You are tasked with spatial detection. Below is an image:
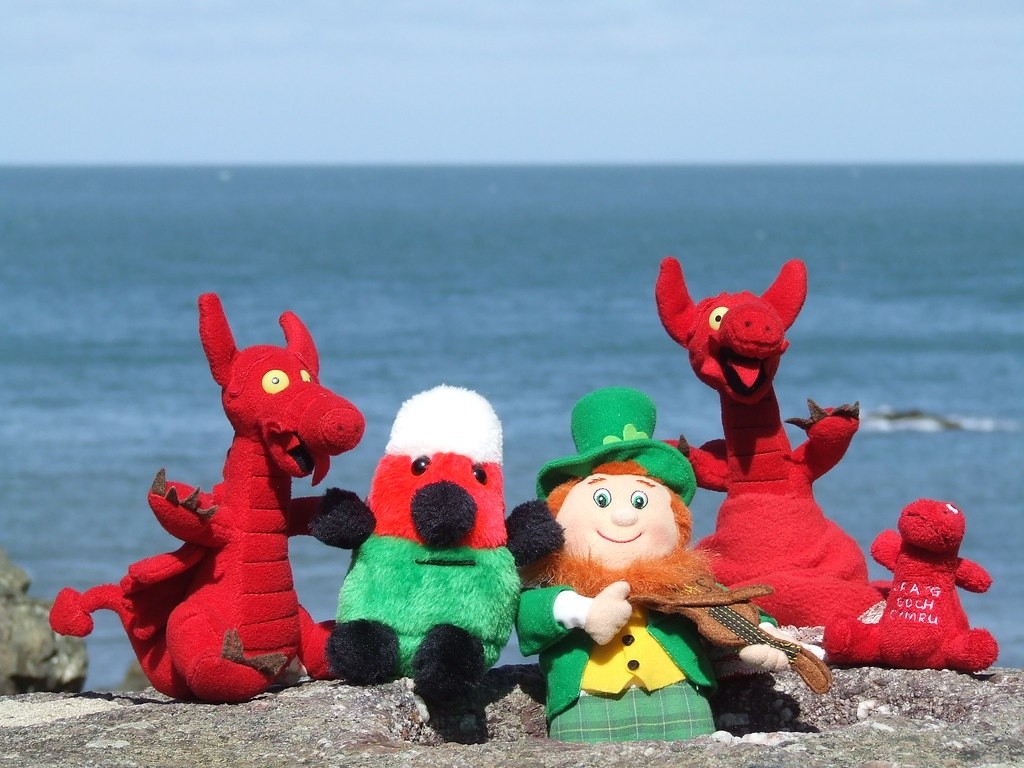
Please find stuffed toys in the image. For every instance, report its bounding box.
[50,293,365,701]
[513,387,831,741]
[655,257,998,672]
[308,383,564,746]
